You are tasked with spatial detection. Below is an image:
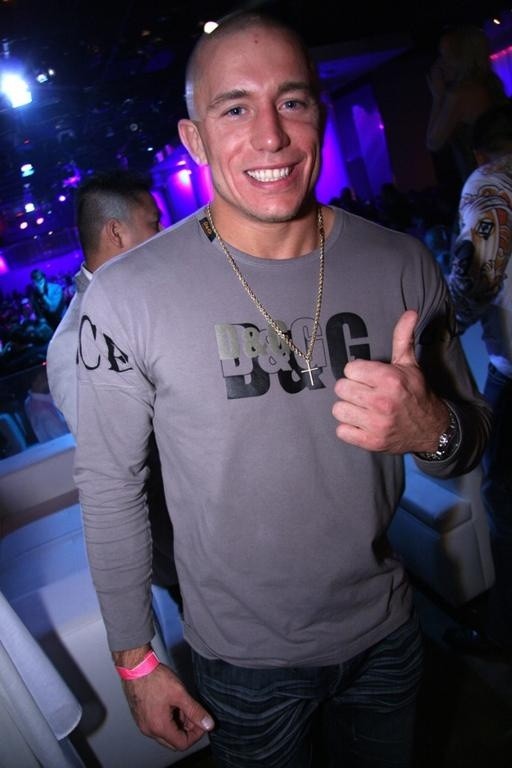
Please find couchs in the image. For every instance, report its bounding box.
[1,429,496,768]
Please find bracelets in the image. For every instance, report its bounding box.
[114,651,161,681]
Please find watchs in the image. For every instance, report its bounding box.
[417,402,460,462]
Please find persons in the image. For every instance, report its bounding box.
[423,21,507,225]
[0,267,78,461]
[444,102,512,662]
[45,169,184,619]
[330,181,453,274]
[71,7,494,767]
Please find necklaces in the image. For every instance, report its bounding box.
[206,202,326,387]
